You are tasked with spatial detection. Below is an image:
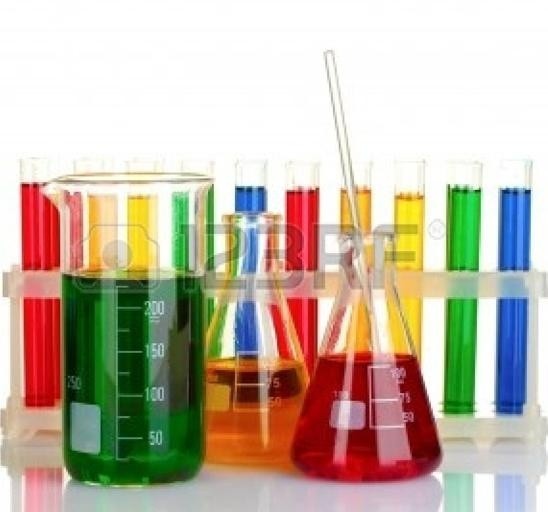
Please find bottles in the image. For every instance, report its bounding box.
[21,157,532,486]
